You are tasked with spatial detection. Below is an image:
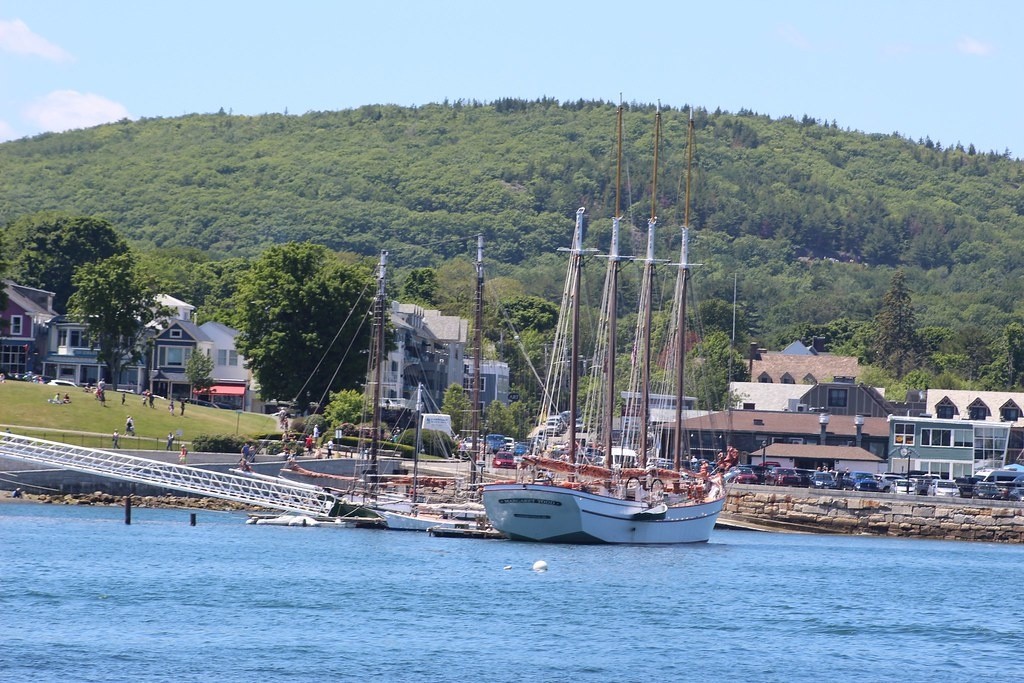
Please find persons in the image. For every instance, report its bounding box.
[142,389,155,409]
[112,429,121,449]
[0,373,5,381]
[392,432,398,442]
[279,406,288,430]
[168,399,186,416]
[94,378,106,406]
[241,443,255,463]
[14,371,33,382]
[814,466,850,479]
[281,424,321,461]
[179,445,188,464]
[121,391,126,405]
[166,431,174,451]
[54,392,70,404]
[123,415,136,437]
[327,438,333,459]
[691,455,697,462]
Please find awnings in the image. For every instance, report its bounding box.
[193,386,246,397]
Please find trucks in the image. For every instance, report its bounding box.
[973,468,1024,482]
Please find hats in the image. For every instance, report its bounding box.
[114,428,118,431]
[181,445,185,447]
[127,415,130,417]
[315,425,319,427]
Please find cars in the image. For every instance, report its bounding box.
[973,482,1024,501]
[844,471,877,491]
[486,411,601,468]
[727,465,846,490]
[48,380,78,387]
[955,477,977,498]
[647,457,716,473]
[907,474,941,494]
[873,474,905,493]
[890,479,917,495]
[927,479,961,497]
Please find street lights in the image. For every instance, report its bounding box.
[906,450,912,495]
[762,440,766,484]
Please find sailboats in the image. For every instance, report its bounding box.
[338,381,494,533]
[227,233,486,519]
[482,91,725,546]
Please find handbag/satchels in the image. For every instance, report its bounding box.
[127,423,131,427]
